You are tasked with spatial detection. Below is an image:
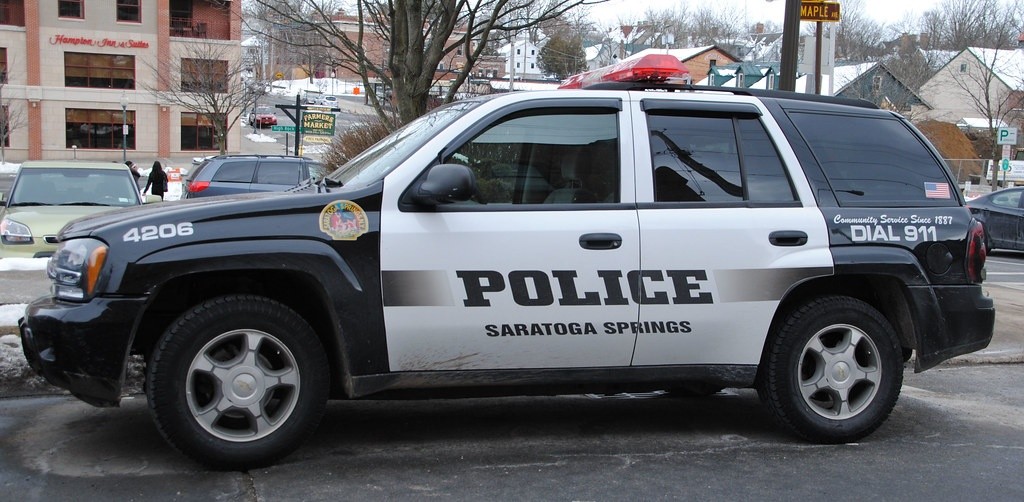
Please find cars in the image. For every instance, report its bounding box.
[320,96,339,112]
[249,106,277,128]
[0,159,162,255]
[964,187,1024,254]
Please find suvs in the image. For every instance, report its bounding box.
[10,49,996,445]
[179,155,334,199]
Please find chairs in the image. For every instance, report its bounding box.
[91,183,112,198]
[65,188,85,197]
[39,180,56,196]
[543,147,596,204]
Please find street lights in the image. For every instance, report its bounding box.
[121,90,129,162]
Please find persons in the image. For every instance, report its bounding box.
[141,160,168,201]
[125,160,140,195]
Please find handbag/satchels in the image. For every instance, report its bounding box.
[163,171,167,192]
